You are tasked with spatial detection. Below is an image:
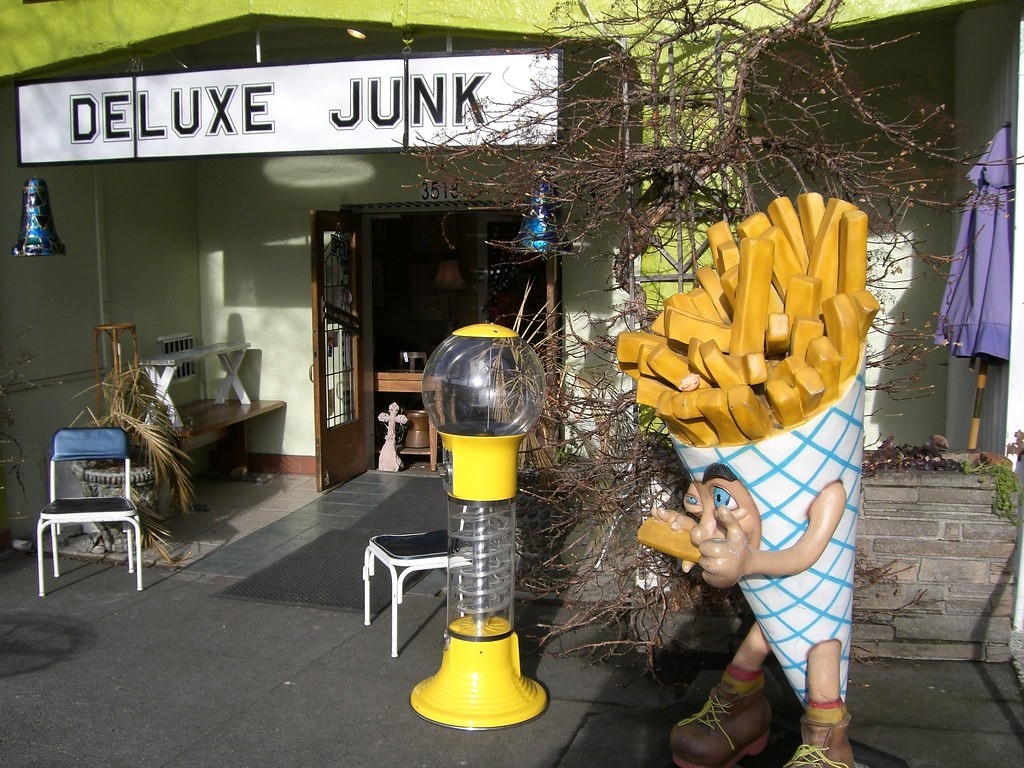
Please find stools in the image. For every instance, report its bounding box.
[400,351,428,372]
[94,322,137,417]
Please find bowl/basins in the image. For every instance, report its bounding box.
[405,409,429,431]
[404,431,432,448]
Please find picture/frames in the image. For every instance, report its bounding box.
[409,258,452,322]
[409,214,462,256]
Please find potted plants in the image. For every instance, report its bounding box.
[61,362,198,567]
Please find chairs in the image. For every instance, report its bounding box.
[361,506,467,658]
[36,427,144,597]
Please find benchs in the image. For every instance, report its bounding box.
[137,342,251,427]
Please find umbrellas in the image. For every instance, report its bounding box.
[932,122,1012,455]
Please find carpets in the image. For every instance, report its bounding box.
[208,478,446,615]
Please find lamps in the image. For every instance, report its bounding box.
[514,159,576,256]
[430,259,468,335]
[11,168,68,258]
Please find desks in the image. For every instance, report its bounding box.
[375,369,441,471]
[171,399,285,513]
